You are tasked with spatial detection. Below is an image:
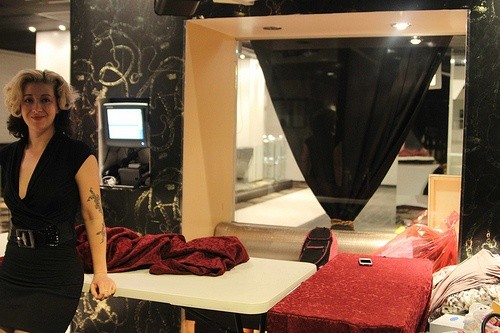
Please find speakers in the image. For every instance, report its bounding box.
[153,0,202,17]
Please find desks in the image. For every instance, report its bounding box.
[268,252,434,332]
[83,255,317,333]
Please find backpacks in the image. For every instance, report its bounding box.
[300,227,339,269]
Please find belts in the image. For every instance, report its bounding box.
[6,225,75,249]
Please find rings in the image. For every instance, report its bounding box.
[104,290,108,292]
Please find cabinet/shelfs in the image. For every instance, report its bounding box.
[395,156,440,209]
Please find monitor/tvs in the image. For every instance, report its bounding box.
[103,102,148,148]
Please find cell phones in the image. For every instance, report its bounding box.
[359,258,372,265]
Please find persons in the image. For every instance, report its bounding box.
[0,67,117,333]
